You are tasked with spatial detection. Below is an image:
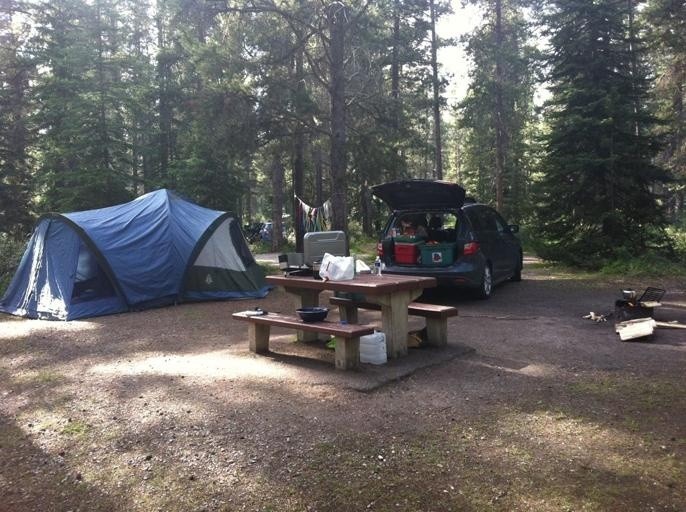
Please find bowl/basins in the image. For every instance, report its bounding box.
[296,307,329,322]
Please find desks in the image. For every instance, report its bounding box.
[266,272,437,358]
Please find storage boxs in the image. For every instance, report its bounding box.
[394,240,426,263]
[418,242,456,266]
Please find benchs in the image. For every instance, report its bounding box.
[329,296,458,348]
[232,311,374,371]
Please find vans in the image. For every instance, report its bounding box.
[370,180,524,299]
[249,222,287,245]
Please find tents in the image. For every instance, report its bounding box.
[0,187,272,320]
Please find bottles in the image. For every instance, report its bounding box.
[374,255,382,278]
